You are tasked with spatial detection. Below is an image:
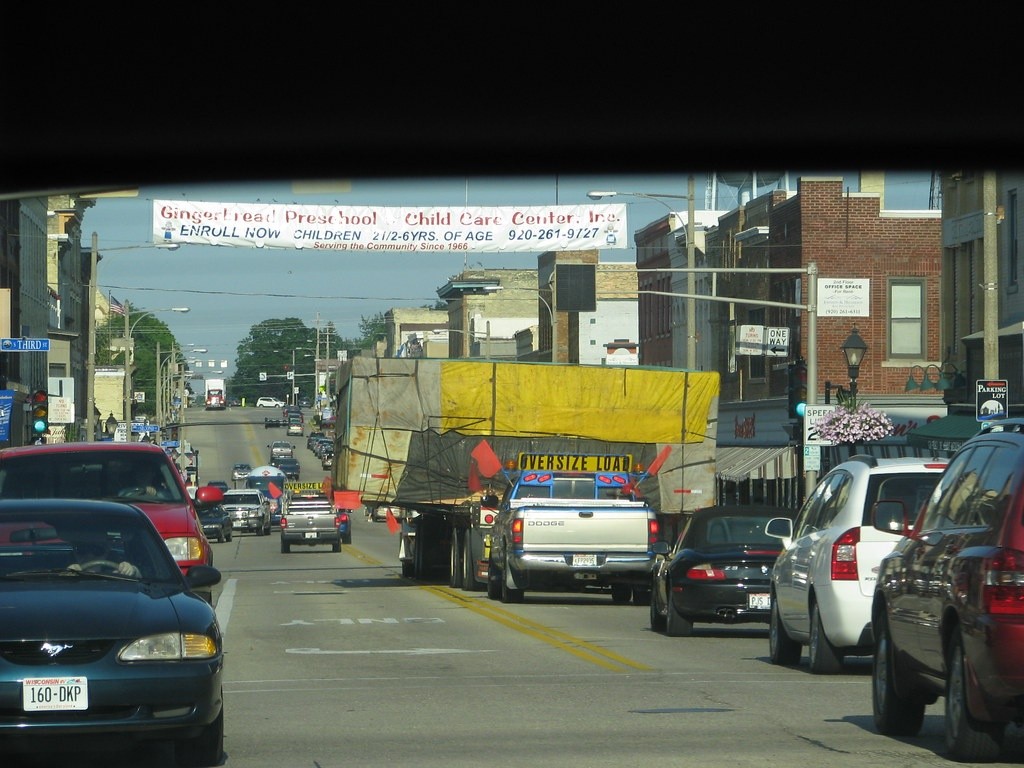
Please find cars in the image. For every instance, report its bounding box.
[283,405,304,436]
[298,396,312,409]
[231,463,252,481]
[0,441,224,768]
[280,490,354,554]
[306,432,336,470]
[650,505,799,637]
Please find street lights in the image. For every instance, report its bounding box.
[482,264,556,361]
[433,319,491,360]
[274,322,329,408]
[86,230,180,442]
[155,341,208,425]
[586,177,696,371]
[124,300,190,443]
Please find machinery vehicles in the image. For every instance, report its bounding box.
[244,466,289,522]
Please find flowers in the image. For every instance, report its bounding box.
[814,390,895,446]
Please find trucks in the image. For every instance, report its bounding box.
[398,503,501,597]
[204,378,227,410]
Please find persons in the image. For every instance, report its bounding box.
[118,457,156,497]
[140,432,158,445]
[64,529,142,580]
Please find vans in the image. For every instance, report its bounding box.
[267,440,301,480]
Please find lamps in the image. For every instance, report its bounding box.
[920,364,954,391]
[937,363,961,390]
[904,365,940,392]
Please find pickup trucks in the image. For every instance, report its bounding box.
[480,469,660,603]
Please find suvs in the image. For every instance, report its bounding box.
[765,454,949,676]
[193,488,272,543]
[871,417,1024,761]
[256,396,286,408]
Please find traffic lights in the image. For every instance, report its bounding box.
[30,387,49,434]
[170,423,179,441]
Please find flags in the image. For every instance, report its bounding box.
[646,445,672,478]
[471,440,503,478]
[322,476,365,509]
[469,462,482,492]
[385,508,401,535]
[268,481,283,499]
[622,480,641,498]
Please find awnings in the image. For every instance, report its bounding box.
[906,415,984,452]
[716,447,796,483]
